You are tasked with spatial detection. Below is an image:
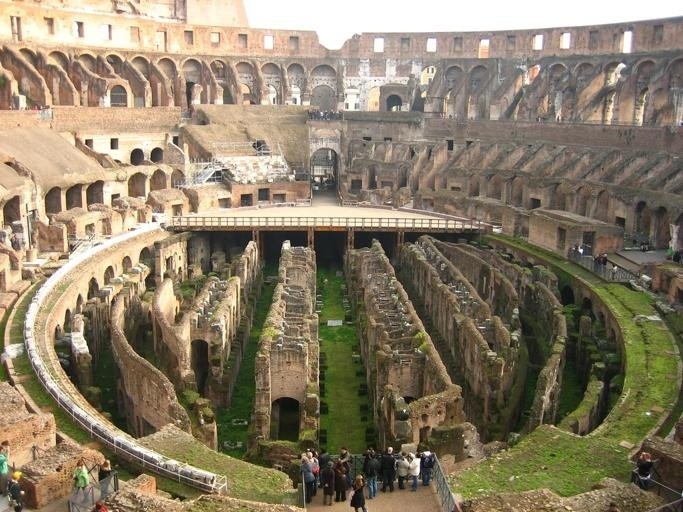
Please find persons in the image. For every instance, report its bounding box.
[299,446,436,511]
[72,459,91,505]
[6,471,24,512]
[0,440,9,496]
[636,451,652,491]
[97,461,114,499]
[576,245,617,280]
